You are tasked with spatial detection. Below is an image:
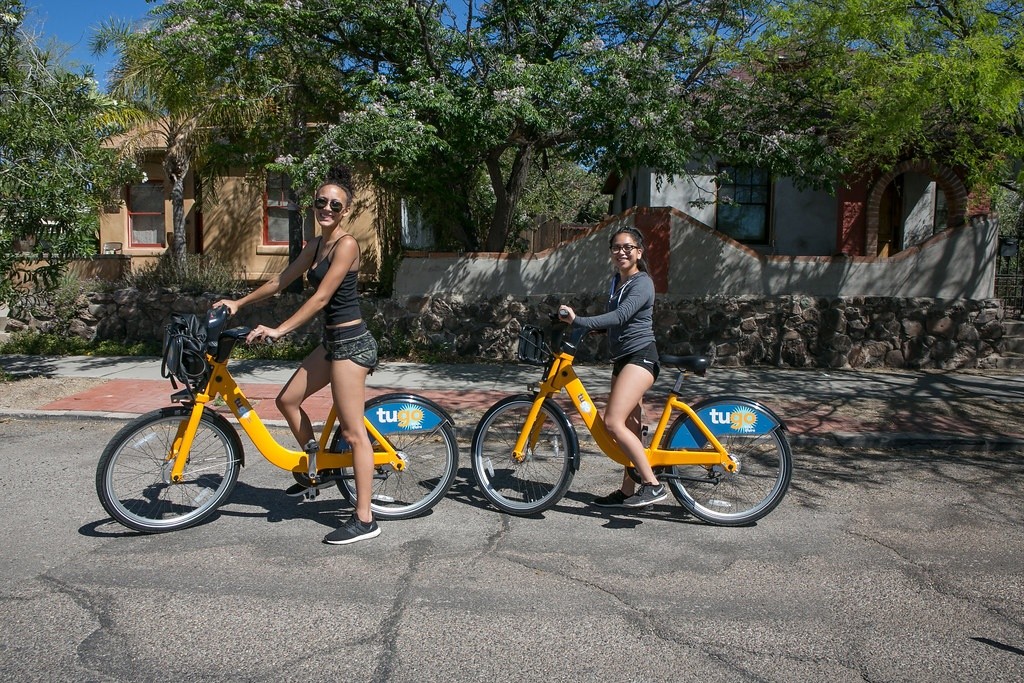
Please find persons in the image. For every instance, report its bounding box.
[211,182,382,545]
[557,225,669,509]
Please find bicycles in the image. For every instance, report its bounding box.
[470,313,793,527]
[96,305,462,534]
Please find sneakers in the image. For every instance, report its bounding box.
[285,471,336,497]
[623,482,667,506]
[324,512,381,544]
[595,489,633,508]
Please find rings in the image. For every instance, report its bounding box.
[253,328,257,332]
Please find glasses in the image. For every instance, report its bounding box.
[609,246,638,253]
[314,198,345,213]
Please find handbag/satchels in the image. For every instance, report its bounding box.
[161,313,208,406]
